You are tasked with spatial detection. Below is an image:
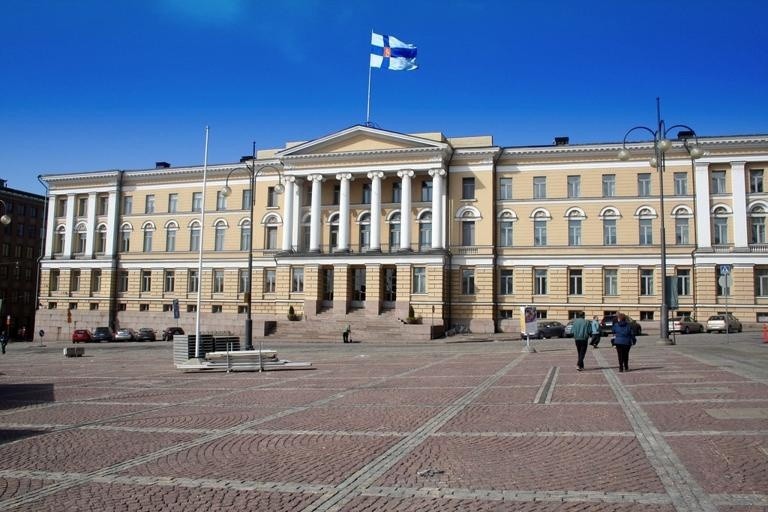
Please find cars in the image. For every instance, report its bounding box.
[668,317,704,335]
[71,327,157,343]
[520,313,641,340]
[162,327,184,341]
[706,314,742,333]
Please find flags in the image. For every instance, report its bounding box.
[370,33,420,72]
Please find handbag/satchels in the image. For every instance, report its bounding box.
[630,334,636,345]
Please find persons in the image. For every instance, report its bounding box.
[613,311,637,372]
[17,327,21,341]
[21,326,27,342]
[342,322,351,343]
[0,330,9,354]
[589,314,600,348]
[570,311,592,370]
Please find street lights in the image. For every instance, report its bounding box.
[221,140,284,349]
[618,96,704,338]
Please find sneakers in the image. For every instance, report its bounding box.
[589,342,599,348]
[576,365,585,371]
